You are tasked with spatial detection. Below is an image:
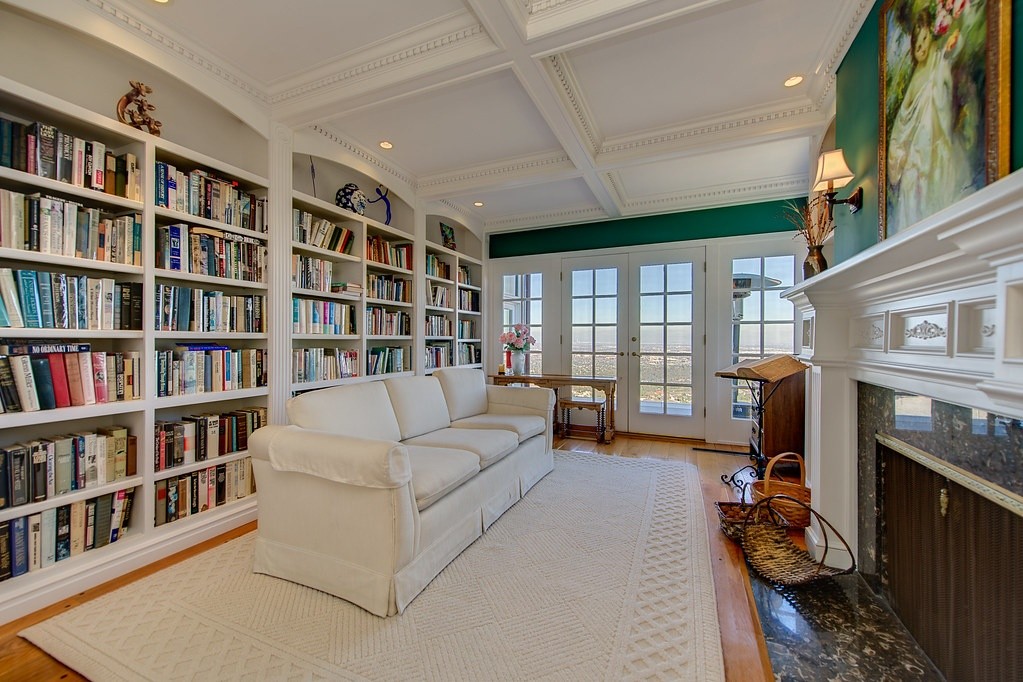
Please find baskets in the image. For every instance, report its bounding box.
[715,500,791,539]
[752,453,811,528]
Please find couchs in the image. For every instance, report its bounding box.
[246,368,556,618]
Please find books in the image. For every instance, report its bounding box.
[155,162,268,234]
[156,284,268,332]
[715,353,808,382]
[156,223,268,282]
[292,254,363,296]
[0,339,141,582]
[425,341,481,368]
[458,265,470,285]
[458,289,479,311]
[367,235,412,270]
[425,314,477,339]
[367,272,412,303]
[0,268,142,331]
[366,346,412,375]
[426,254,450,280]
[0,117,141,202]
[0,189,141,265]
[293,209,355,253]
[366,306,411,335]
[292,298,357,334]
[292,347,360,382]
[426,279,455,308]
[155,343,268,525]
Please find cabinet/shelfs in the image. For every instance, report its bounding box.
[0,74,480,623]
[750,370,805,470]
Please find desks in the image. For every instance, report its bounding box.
[487,374,616,444]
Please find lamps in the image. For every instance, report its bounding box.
[813,149,864,222]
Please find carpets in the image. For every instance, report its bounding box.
[17,449,726,682]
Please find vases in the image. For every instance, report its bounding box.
[803,245,827,281]
[505,349,516,376]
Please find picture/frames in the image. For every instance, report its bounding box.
[877,0,1012,242]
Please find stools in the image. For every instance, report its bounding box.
[560,396,607,444]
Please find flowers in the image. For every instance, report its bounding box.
[498,323,536,354]
